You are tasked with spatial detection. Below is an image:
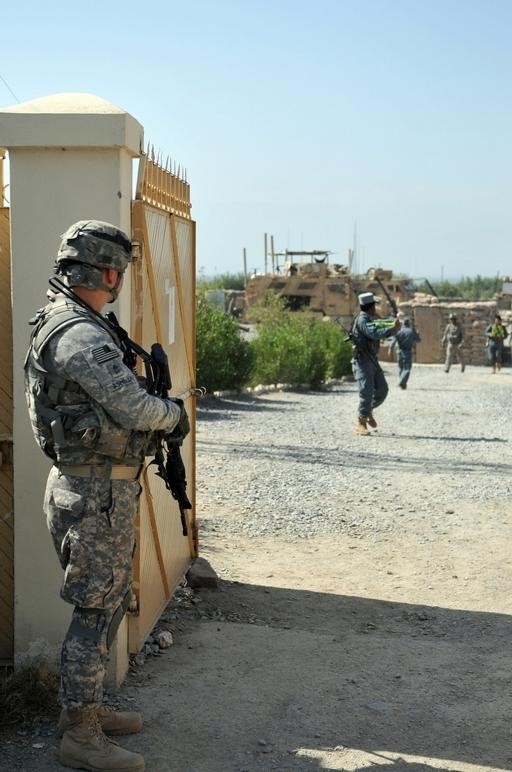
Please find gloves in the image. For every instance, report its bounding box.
[171,397,191,442]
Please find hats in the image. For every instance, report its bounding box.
[358,291,381,306]
[446,312,460,320]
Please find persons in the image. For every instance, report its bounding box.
[21,221,189,770]
[386,317,423,390]
[440,313,467,374]
[351,292,400,432]
[485,315,507,373]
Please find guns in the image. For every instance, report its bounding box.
[145,344,192,536]
[335,318,384,376]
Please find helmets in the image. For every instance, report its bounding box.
[56,218,133,273]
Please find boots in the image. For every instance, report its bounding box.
[59,704,145,772]
[355,414,371,436]
[59,700,144,737]
[366,414,378,428]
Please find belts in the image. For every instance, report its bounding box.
[52,459,146,483]
[398,345,412,351]
